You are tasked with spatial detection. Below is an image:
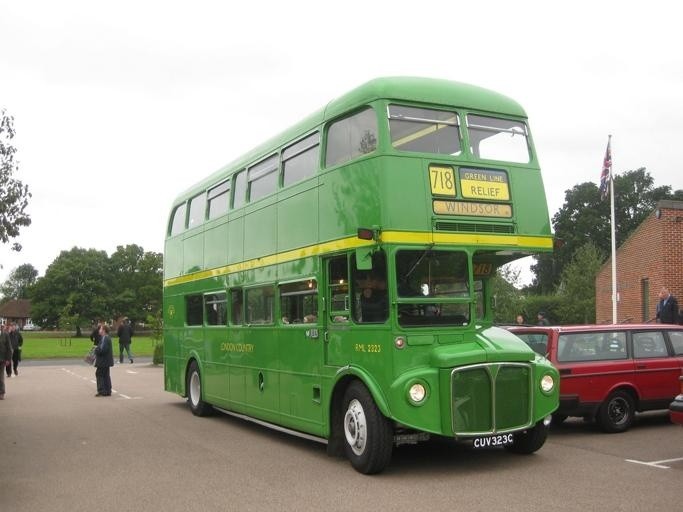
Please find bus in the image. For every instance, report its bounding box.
[160,73,564,478]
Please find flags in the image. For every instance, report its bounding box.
[597,136,611,201]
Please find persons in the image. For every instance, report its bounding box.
[537,310,550,326]
[90,320,105,366]
[660,287,679,325]
[358,284,385,322]
[655,292,663,315]
[1,320,24,400]
[366,256,441,318]
[92,325,114,396]
[117,319,134,362]
[515,315,526,326]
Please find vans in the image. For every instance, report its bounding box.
[502,321,683,433]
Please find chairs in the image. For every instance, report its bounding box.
[607,337,623,354]
[638,336,657,354]
[531,343,547,355]
